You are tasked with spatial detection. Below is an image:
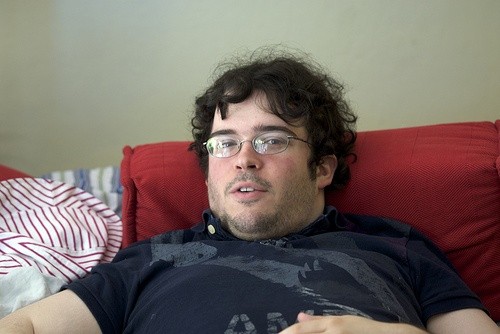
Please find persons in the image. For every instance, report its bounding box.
[0,44,500,334]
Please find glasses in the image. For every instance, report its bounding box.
[202,130,313,158]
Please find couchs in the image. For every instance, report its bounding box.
[120,119,500,324]
